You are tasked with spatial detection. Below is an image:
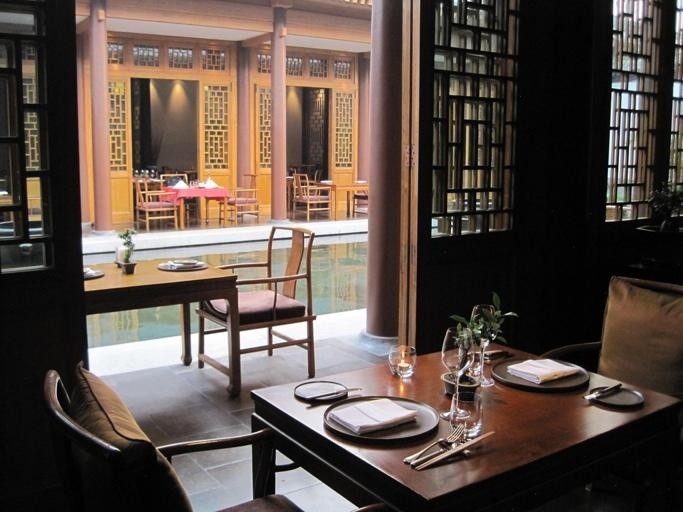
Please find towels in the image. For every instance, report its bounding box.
[328,398,418,435]
[84,268,94,276]
[507,359,578,384]
[167,261,202,269]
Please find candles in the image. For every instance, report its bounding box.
[396,352,412,372]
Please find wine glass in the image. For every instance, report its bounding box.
[439,327,474,420]
[470,305,495,388]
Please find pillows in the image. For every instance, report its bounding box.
[66,363,199,511]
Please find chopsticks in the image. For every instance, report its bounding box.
[413,431,494,471]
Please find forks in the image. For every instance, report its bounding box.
[402,423,464,464]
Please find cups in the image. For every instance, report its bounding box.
[189,180,193,187]
[388,345,416,378]
[449,391,481,438]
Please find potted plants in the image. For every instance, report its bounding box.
[634,180,683,268]
[114,229,136,274]
[440,292,519,400]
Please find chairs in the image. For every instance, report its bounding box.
[541,275,682,511]
[42,360,388,512]
[353,180,368,213]
[292,174,332,221]
[136,179,177,232]
[219,174,260,225]
[199,226,316,395]
[160,174,188,220]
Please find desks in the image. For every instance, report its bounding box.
[160,186,227,227]
[313,182,368,214]
[133,176,166,215]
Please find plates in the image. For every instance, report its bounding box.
[490,358,589,394]
[157,262,208,271]
[83,270,104,278]
[323,395,439,446]
[589,387,644,409]
[355,181,366,183]
[286,176,294,178]
[320,180,331,183]
[293,380,347,404]
